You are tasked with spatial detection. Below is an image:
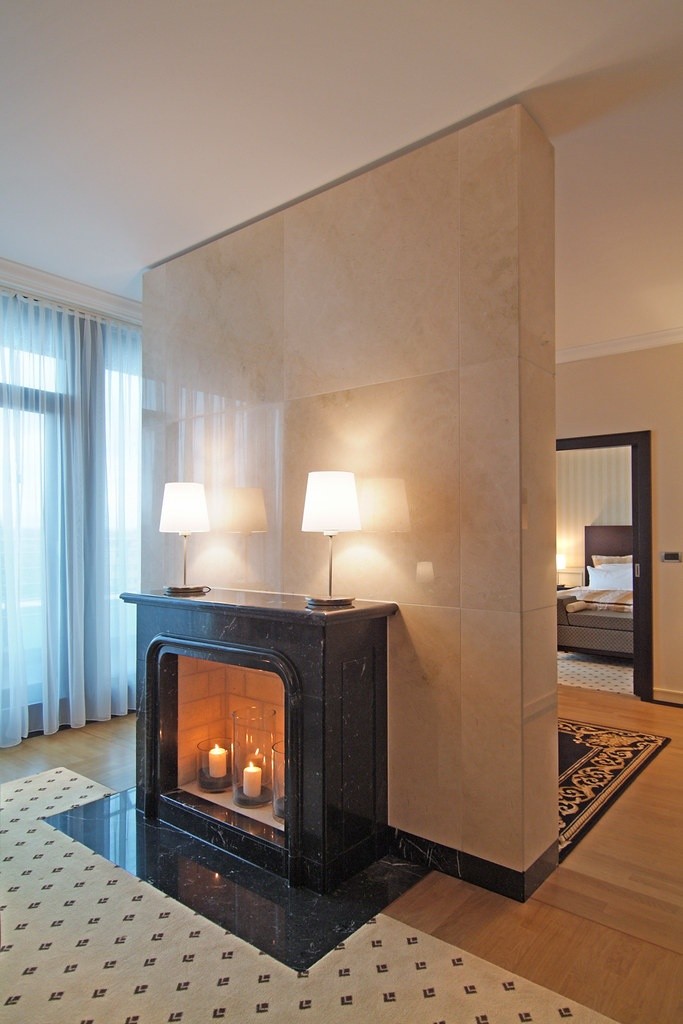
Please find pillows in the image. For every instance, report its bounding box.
[587,566,633,590]
[594,563,633,572]
[591,554,633,567]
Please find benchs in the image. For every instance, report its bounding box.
[557,595,634,659]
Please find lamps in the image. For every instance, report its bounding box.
[300,470,363,607]
[222,487,269,590]
[159,482,211,593]
[556,553,567,587]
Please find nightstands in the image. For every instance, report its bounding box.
[557,585,581,591]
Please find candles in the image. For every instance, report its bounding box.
[232,759,275,808]
[196,736,242,793]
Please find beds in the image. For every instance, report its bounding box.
[557,526,634,613]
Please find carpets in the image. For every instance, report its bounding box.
[557,716,672,865]
